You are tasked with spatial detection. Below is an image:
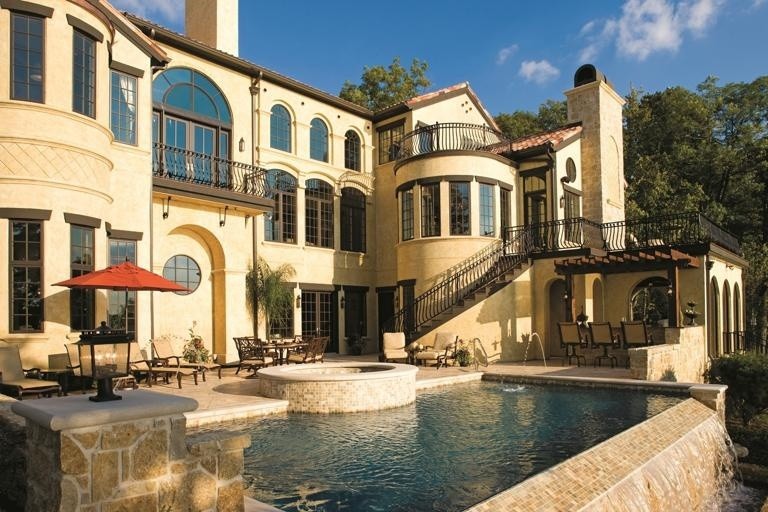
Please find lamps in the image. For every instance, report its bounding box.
[72,321,135,401]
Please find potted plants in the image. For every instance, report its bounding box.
[684,300,703,325]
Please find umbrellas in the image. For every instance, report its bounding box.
[51,255,194,335]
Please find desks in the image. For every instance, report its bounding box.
[253,340,310,365]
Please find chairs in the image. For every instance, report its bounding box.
[382,332,459,369]
[232,335,330,377]
[1,334,223,402]
[558,322,653,369]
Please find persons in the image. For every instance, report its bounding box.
[96,321,110,330]
